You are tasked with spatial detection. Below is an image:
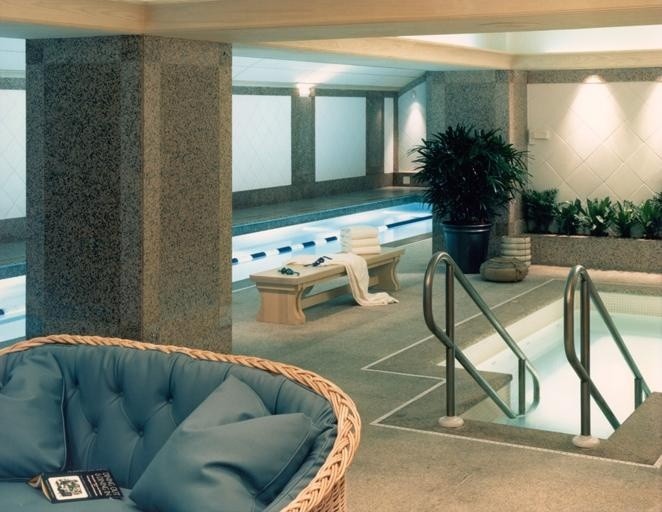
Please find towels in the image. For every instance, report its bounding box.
[290,222,397,309]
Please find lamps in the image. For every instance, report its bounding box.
[298,86,313,101]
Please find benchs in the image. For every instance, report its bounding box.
[247,247,407,325]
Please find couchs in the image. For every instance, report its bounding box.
[0,334,360,512]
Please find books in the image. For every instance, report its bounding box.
[25,467,125,503]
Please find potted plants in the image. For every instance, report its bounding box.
[407,132,662,274]
